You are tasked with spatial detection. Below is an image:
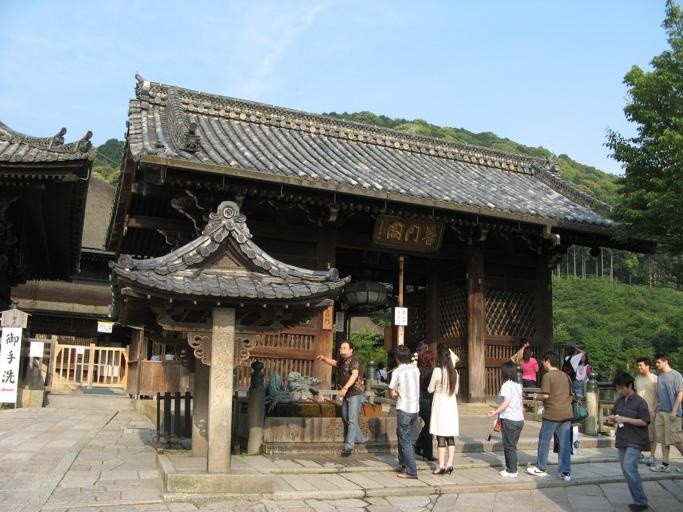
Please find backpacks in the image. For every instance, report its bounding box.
[562,355,576,382]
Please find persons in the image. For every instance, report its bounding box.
[634,353,683,472]
[608,372,652,511]
[388,341,460,478]
[317,341,366,454]
[488,361,524,479]
[520,344,591,482]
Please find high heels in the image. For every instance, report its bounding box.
[398,445,453,478]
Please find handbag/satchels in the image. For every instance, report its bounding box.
[553,426,579,455]
[494,413,502,432]
[572,399,589,423]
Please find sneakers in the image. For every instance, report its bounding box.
[499,467,570,480]
[629,454,683,512]
